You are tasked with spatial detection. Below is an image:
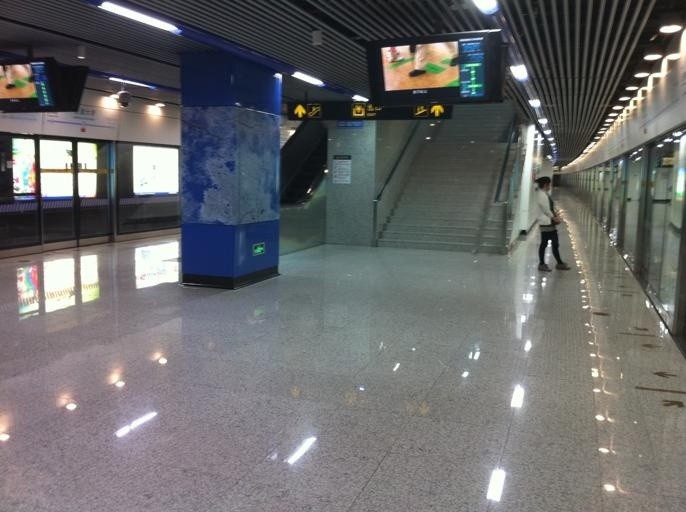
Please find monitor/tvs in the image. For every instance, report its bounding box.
[0,57,64,113]
[61,64,89,112]
[367,29,502,108]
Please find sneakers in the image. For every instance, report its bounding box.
[557,263,571,270]
[450,56,460,65]
[386,48,404,62]
[539,264,552,271]
[410,70,425,76]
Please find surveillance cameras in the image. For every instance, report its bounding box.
[118,91,132,108]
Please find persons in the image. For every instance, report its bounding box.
[533,176,571,272]
[408,42,460,77]
[387,47,404,63]
